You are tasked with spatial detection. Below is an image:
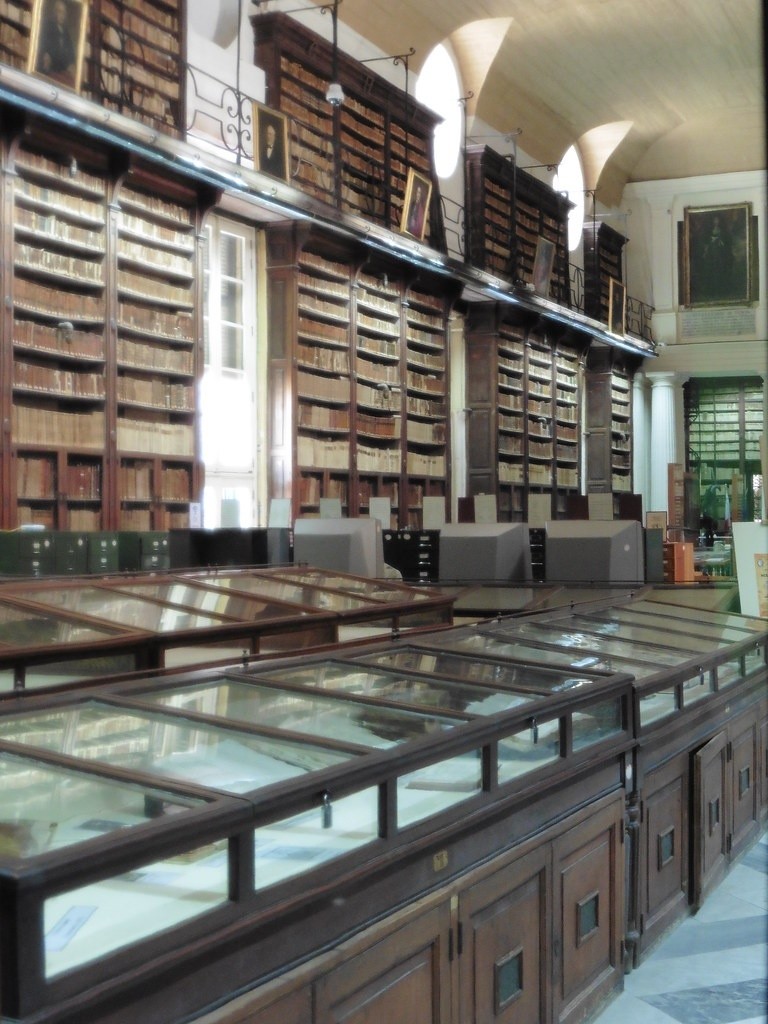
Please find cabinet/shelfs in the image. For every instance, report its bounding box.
[252,100,292,186]
[179,667,767,1024]
[662,541,695,585]
[25,0,89,97]
[0,109,634,580]
[399,166,434,246]
[683,201,753,308]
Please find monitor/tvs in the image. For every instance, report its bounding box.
[294,517,644,586]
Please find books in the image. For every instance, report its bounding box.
[405,756,502,792]
[463,692,599,753]
[122,804,229,866]
[0,0,634,531]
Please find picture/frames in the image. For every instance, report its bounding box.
[608,275,627,338]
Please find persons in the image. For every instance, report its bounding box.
[260,125,283,178]
[39,1,76,89]
[406,187,425,240]
[612,291,622,324]
